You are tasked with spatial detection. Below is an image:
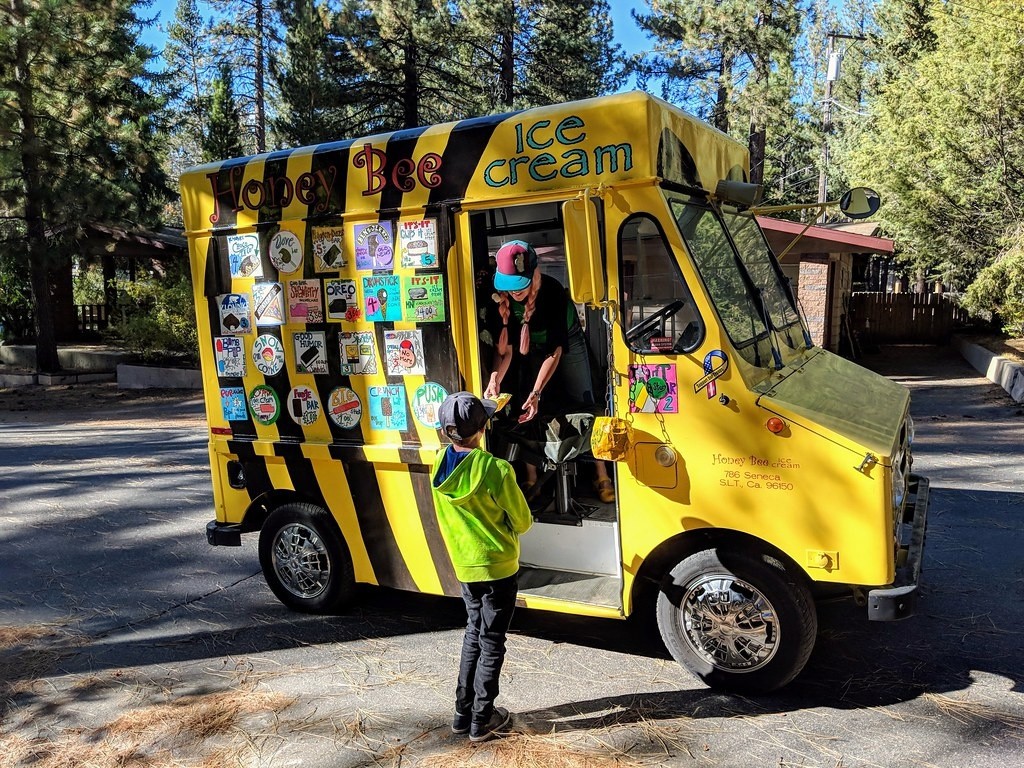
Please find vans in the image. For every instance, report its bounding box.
[176,89,930,696]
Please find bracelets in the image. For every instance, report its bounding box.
[533,390,541,401]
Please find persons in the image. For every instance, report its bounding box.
[430,392,534,743]
[484,239,616,504]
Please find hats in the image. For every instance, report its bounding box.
[436,391,497,439]
[492,240,537,291]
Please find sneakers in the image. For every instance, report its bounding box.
[451,711,472,733]
[469,707,510,742]
